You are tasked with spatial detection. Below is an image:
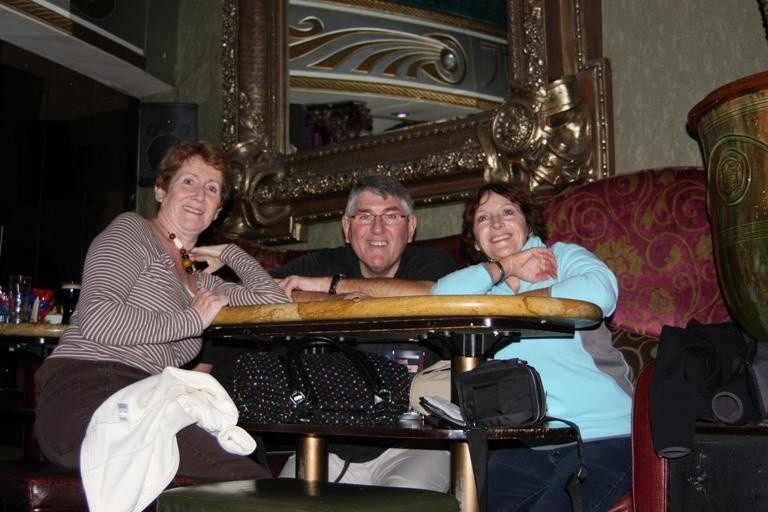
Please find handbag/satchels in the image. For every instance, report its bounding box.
[232,332,411,427]
[454,356,547,429]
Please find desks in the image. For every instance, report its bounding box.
[2,322,68,344]
[213,295,603,510]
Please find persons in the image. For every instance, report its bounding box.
[431,179,634,512]
[278,175,451,496]
[33,139,290,482]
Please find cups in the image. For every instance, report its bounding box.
[10,275,32,323]
[61,284,81,323]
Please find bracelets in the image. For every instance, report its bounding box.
[329,273,346,295]
[490,256,506,280]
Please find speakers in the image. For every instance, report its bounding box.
[138,102,198,187]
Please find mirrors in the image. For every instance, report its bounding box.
[219,1,614,240]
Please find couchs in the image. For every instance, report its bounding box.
[198,173,724,511]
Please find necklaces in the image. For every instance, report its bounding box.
[158,220,196,277]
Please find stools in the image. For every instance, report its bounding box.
[150,478,459,510]
[2,468,88,510]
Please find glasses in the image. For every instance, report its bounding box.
[347,210,408,226]
